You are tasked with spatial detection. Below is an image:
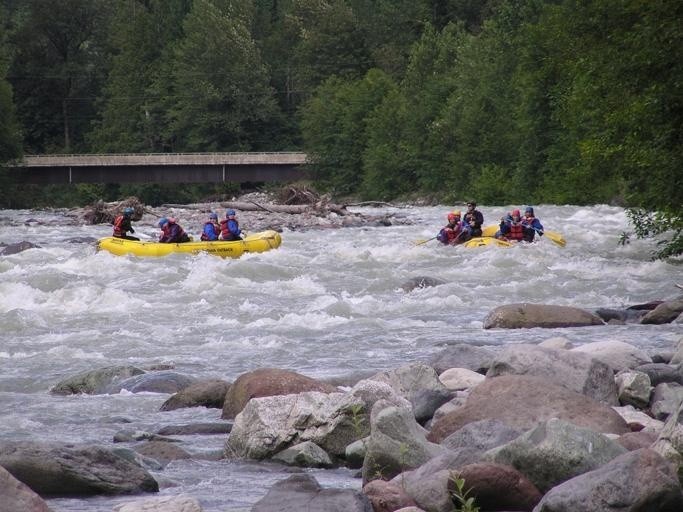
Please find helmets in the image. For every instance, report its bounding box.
[452,209,460,216]
[208,213,219,219]
[158,218,168,227]
[447,213,455,220]
[123,207,135,216]
[225,210,235,216]
[466,201,476,209]
[511,210,520,216]
[525,206,533,213]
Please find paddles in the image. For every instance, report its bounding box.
[408,237,439,250]
[506,218,562,241]
[553,239,567,247]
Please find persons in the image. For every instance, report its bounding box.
[498,208,532,242]
[439,213,472,245]
[436,209,469,242]
[157,217,190,243]
[109,208,140,241]
[199,213,221,241]
[218,209,245,241]
[462,200,484,237]
[517,205,543,239]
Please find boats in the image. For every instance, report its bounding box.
[95,230,281,259]
[462,224,513,248]
